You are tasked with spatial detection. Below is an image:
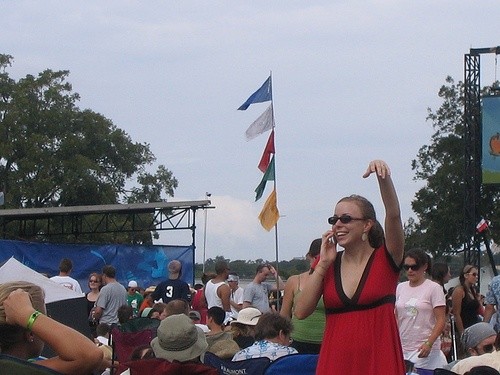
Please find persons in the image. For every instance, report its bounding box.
[0,258,298,375]
[294,160,405,375]
[431,263,500,375]
[280,239,325,355]
[394,248,449,369]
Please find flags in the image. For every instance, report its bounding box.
[258,131,276,173]
[259,190,279,231]
[477,219,488,233]
[246,105,275,142]
[254,155,275,200]
[237,75,272,110]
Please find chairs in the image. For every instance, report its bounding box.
[108,317,319,375]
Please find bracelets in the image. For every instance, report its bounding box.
[26,311,42,331]
[425,342,432,346]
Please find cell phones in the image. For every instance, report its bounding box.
[331,236,337,244]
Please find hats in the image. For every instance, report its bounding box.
[128,281,137,287]
[168,260,181,279]
[310,239,322,257]
[230,308,261,326]
[150,314,209,362]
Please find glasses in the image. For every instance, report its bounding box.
[474,345,493,352]
[284,333,294,344]
[467,273,479,277]
[89,280,100,283]
[328,216,367,224]
[404,264,423,271]
